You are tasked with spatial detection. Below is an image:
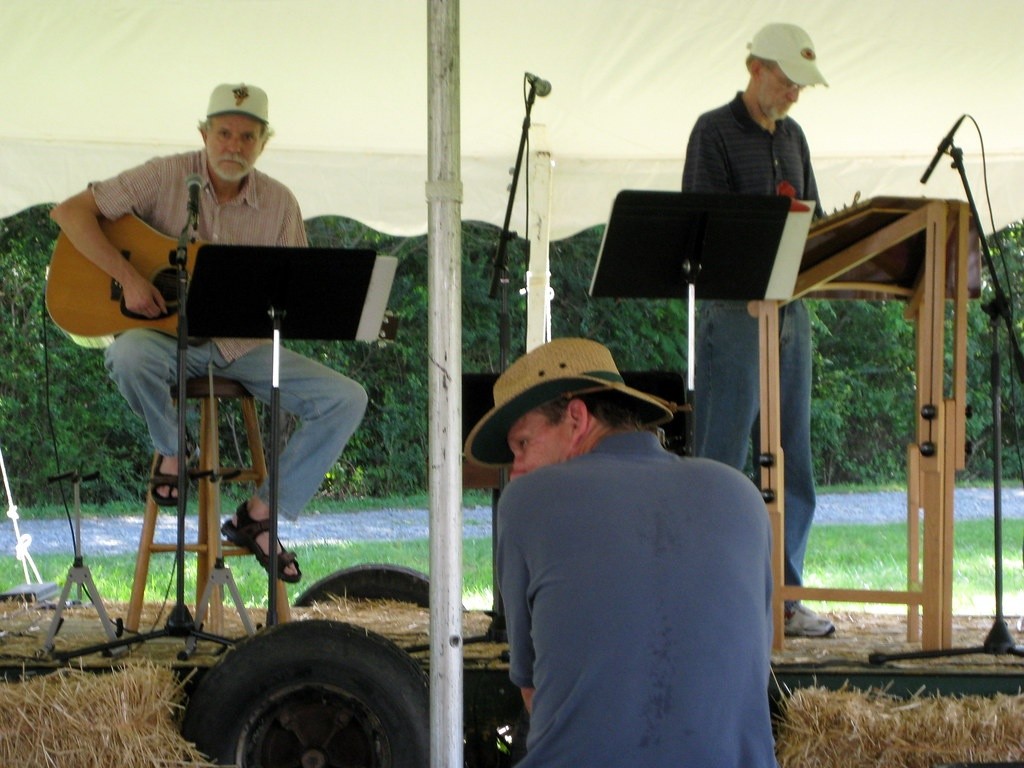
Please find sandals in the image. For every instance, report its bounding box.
[222,501,302,582]
[148,426,199,507]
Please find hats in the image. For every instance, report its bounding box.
[745,23,829,87]
[464,338,673,467]
[205,83,269,124]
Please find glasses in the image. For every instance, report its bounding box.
[765,64,805,90]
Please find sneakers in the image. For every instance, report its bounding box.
[785,603,835,637]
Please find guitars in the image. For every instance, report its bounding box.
[46,209,401,349]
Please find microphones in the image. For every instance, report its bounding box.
[186,174,203,231]
[921,116,965,183]
[526,73,551,96]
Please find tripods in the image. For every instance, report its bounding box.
[55,206,237,666]
[870,143,1024,667]
[402,89,536,655]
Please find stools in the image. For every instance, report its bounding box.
[119,374,290,640]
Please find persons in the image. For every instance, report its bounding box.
[464,339,780,768]
[49,82,367,580]
[680,23,836,637]
